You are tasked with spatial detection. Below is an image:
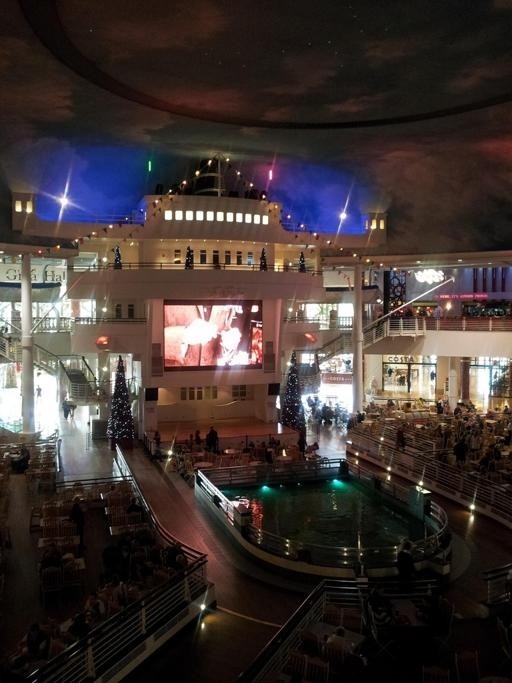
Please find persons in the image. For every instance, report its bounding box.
[321,623,355,662]
[165,304,243,368]
[0,484,188,683]
[71,405,76,418]
[59,398,69,418]
[19,441,30,470]
[183,425,283,462]
[34,386,44,402]
[304,395,511,472]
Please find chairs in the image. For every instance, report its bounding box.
[1,434,61,481]
[172,438,319,483]
[265,594,511,682]
[359,408,511,492]
[1,476,186,682]
